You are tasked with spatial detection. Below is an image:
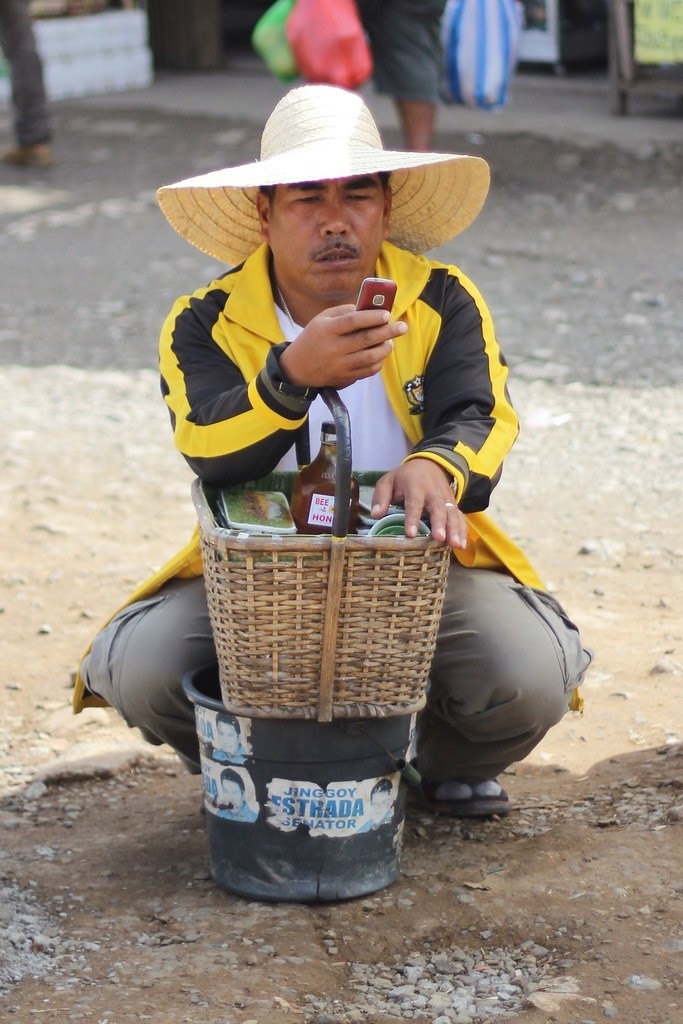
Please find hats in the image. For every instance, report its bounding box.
[155,81,492,266]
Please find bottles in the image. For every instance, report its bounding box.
[290,420,357,537]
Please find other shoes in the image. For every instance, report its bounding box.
[411,774,511,819]
[0,142,52,167]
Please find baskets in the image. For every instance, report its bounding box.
[190,385,452,720]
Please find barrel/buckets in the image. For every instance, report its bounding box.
[184,661,431,901]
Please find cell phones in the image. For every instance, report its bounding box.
[349,278,397,336]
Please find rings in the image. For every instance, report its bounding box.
[445,502,456,507]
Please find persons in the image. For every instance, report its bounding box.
[347,0,444,157]
[72,81,596,824]
[0,0,57,168]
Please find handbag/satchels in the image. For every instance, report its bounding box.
[287,0,372,89]
[438,0,522,109]
[251,0,301,80]
